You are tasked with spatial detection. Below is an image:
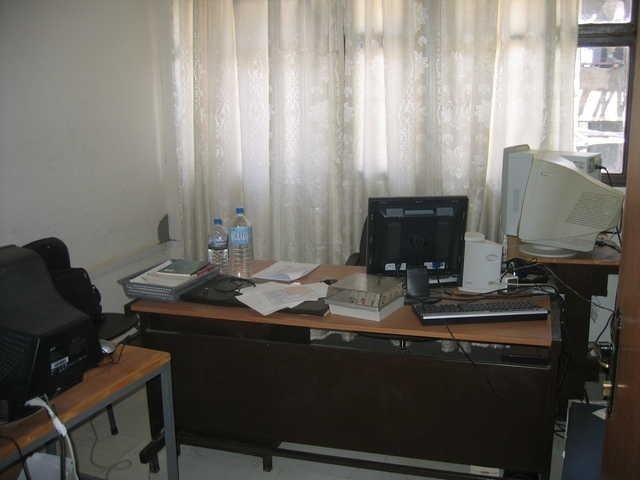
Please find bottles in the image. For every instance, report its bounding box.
[207,217,230,278]
[229,207,257,278]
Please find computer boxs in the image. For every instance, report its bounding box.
[501,144,602,237]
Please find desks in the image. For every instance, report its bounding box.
[507,232,620,405]
[124,256,562,480]
[0,338,179,480]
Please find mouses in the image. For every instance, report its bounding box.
[98,338,116,354]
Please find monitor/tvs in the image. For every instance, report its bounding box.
[365,195,469,303]
[0,244,94,422]
[517,151,626,258]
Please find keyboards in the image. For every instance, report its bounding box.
[411,299,549,325]
[428,274,458,285]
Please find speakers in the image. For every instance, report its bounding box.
[457,240,507,294]
[464,231,485,240]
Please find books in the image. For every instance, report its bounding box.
[116,257,221,302]
[323,271,405,322]
